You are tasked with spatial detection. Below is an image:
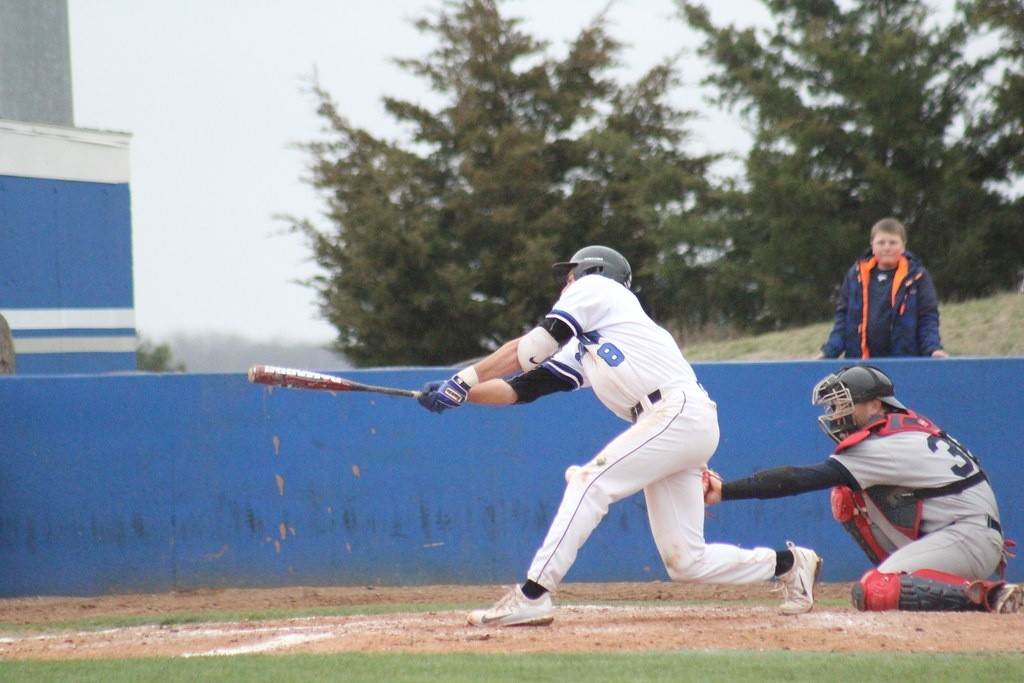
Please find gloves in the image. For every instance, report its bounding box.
[419,374,472,415]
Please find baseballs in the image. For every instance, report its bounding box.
[564,465,581,482]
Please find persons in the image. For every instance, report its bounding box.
[813,218,948,358]
[704,366,1024,613]
[418,245,823,628]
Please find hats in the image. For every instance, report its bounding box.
[827,365,907,410]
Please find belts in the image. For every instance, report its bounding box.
[951,517,1002,536]
[631,389,662,424]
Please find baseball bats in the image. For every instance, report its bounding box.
[248,364,422,398]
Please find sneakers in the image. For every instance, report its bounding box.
[468,584,554,626]
[775,541,823,614]
[995,583,1024,614]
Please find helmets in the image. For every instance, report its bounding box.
[552,245,632,290]
